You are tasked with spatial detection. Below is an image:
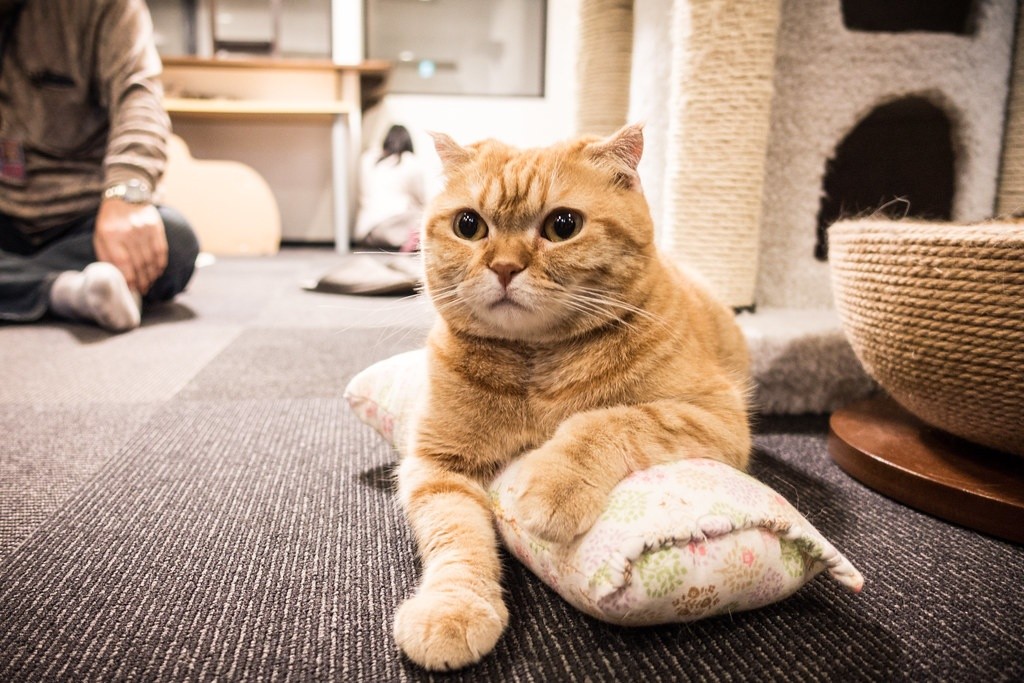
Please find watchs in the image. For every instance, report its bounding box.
[102,179,151,203]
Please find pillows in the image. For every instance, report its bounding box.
[343,348,863,625]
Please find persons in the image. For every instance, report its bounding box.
[0,0,199,332]
[358,123,424,250]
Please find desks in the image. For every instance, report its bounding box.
[161,57,390,255]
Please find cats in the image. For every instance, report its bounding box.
[390,119,760,673]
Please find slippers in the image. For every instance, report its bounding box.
[303,261,415,294]
